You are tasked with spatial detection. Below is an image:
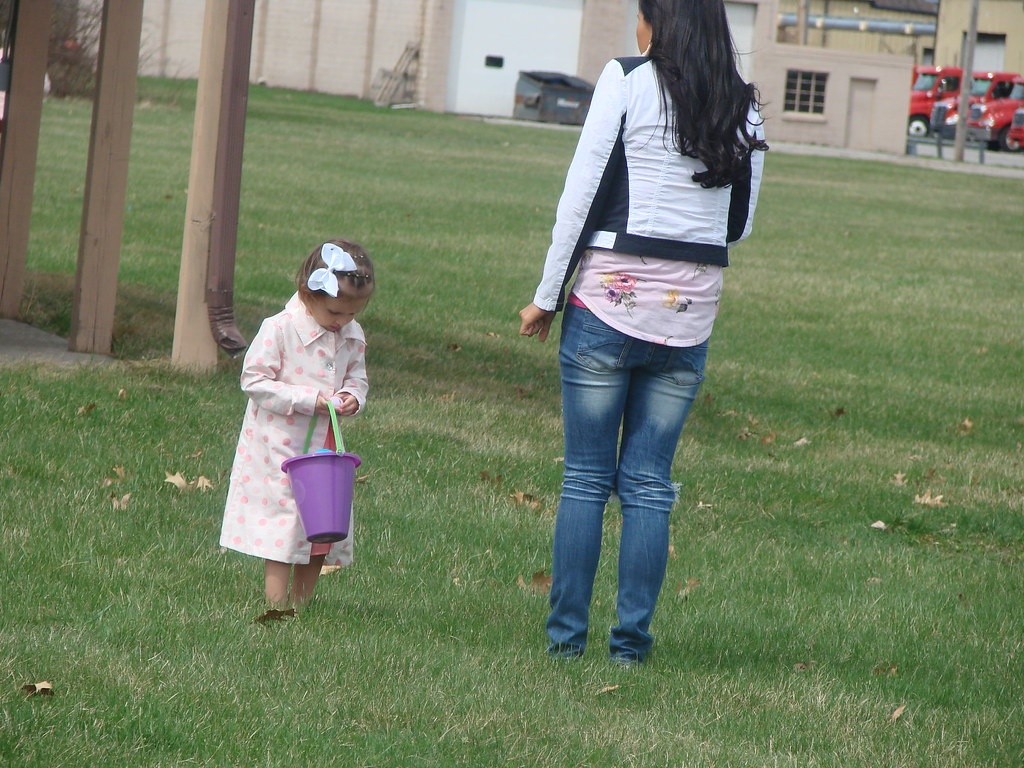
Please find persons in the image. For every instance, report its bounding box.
[518,1,771,667]
[220,239,376,602]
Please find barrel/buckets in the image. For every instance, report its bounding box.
[280,400,361,544]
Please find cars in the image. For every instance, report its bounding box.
[908,66,963,136]
[966,77,1024,152]
[931,70,1019,138]
